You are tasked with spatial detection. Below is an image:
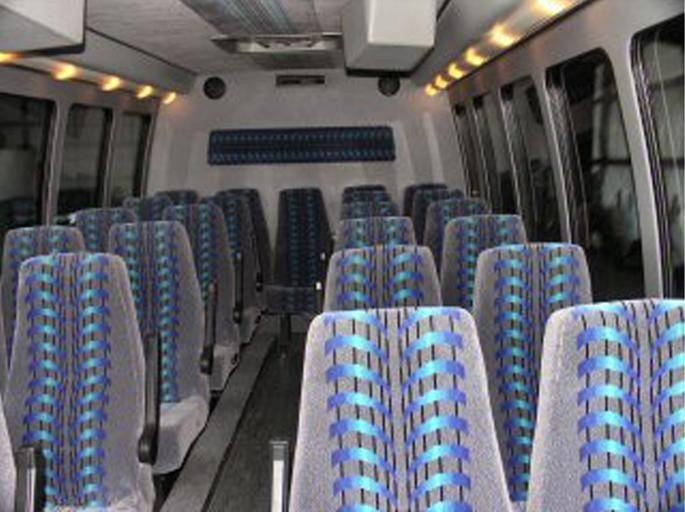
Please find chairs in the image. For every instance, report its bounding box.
[322,183,528,314]
[525,297,683,510]
[472,242,594,511]
[67,207,140,256]
[1,253,163,512]
[0,226,86,374]
[108,220,212,475]
[271,184,335,355]
[122,189,271,393]
[273,305,515,511]
